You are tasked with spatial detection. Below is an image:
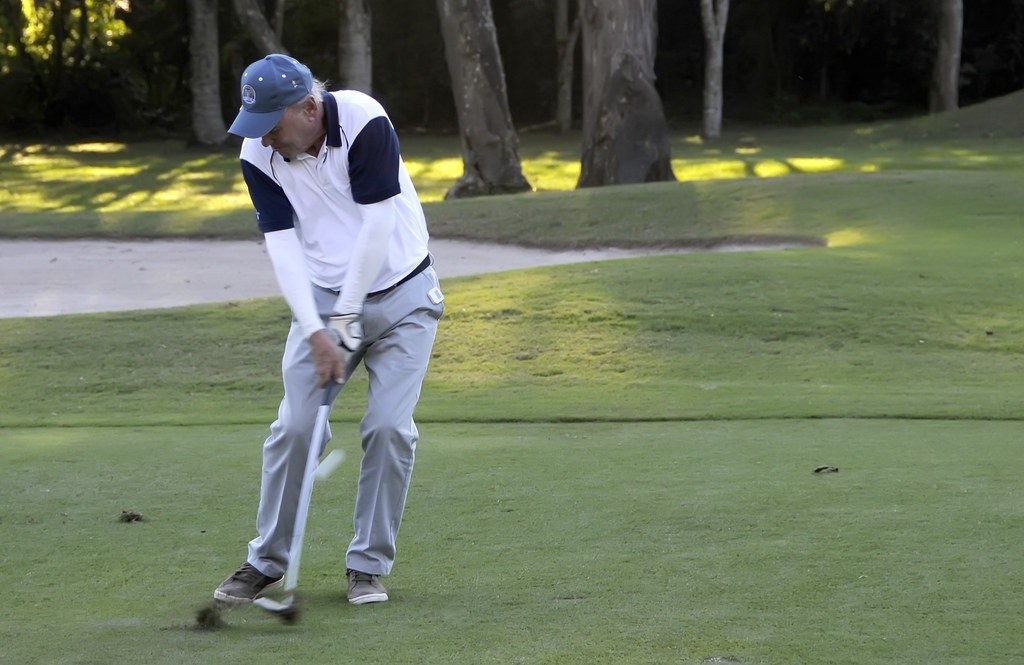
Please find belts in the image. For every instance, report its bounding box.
[306,253,432,301]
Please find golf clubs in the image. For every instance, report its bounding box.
[253,336,339,612]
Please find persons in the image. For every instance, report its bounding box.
[214,54,444,603]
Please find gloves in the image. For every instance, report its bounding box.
[327,312,364,352]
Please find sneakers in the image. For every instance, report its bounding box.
[213,561,285,605]
[345,569,388,605]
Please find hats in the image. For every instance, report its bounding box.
[227,53,314,140]
